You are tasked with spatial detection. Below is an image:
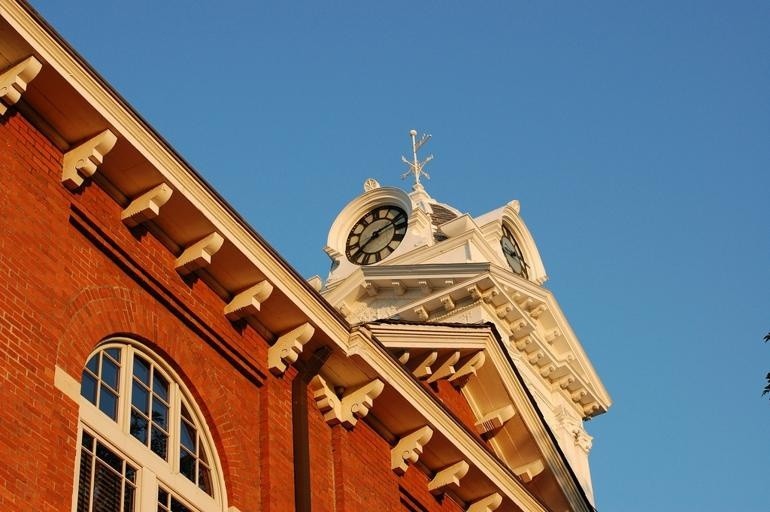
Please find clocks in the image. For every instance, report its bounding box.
[345,204,409,267]
[499,224,530,282]
